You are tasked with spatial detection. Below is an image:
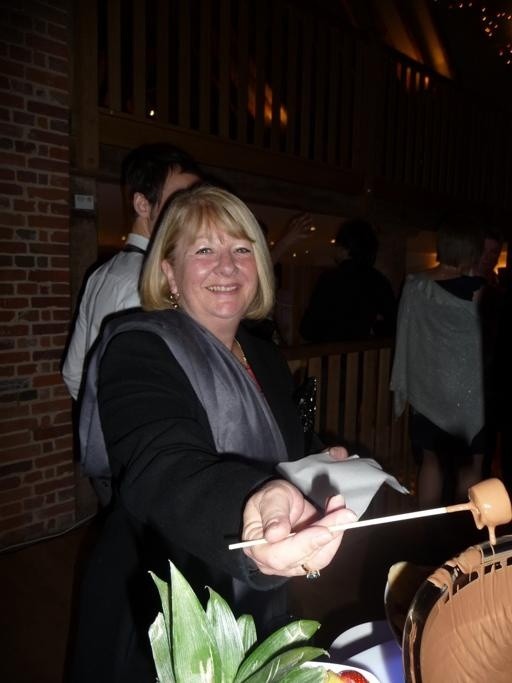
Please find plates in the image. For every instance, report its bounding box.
[301,619,407,683]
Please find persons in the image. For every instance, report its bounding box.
[473,225,503,287]
[298,217,396,343]
[240,209,313,347]
[59,141,205,521]
[56,181,359,683]
[387,212,485,509]
[477,232,512,532]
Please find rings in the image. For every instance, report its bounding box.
[299,562,321,578]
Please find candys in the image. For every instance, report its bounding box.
[228,478,512,552]
